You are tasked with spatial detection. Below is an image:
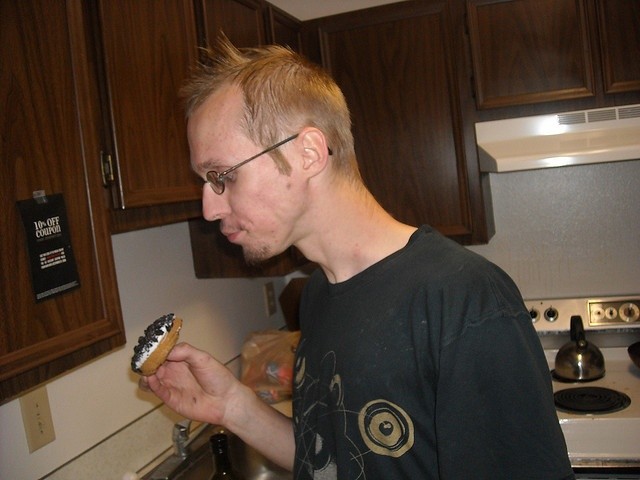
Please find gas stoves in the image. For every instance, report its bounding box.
[539,336,640,415]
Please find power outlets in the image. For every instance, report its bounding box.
[19,387,56,454]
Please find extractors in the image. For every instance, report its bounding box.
[468,91,640,176]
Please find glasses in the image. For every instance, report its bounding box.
[201,134,335,195]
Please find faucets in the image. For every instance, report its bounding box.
[171,417,194,461]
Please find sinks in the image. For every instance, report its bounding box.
[140,422,296,480]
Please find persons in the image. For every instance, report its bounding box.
[140,30,574,480]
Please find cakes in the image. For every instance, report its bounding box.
[131,312,183,377]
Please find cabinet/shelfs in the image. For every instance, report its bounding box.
[299,0,496,247]
[469,0,640,109]
[0,0,127,404]
[87,1,267,210]
[189,7,310,279]
